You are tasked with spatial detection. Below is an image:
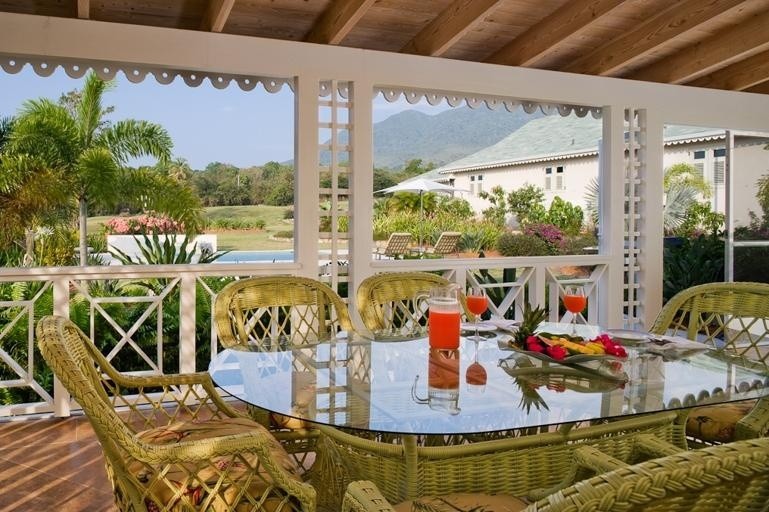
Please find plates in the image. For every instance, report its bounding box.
[460,332,498,339]
[460,322,498,332]
[602,328,656,346]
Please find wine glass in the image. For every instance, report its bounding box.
[563,285,587,337]
[466,339,487,394]
[465,286,488,341]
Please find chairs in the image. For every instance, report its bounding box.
[373,231,462,259]
[36,313,319,510]
[213,274,355,483]
[357,270,476,339]
[337,434,769,511]
[647,281,769,364]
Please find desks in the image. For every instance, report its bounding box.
[206,318,768,437]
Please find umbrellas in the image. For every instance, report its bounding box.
[375,179,471,248]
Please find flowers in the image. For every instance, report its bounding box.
[106,214,187,234]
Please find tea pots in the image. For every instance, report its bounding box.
[413,283,463,350]
[411,348,462,416]
